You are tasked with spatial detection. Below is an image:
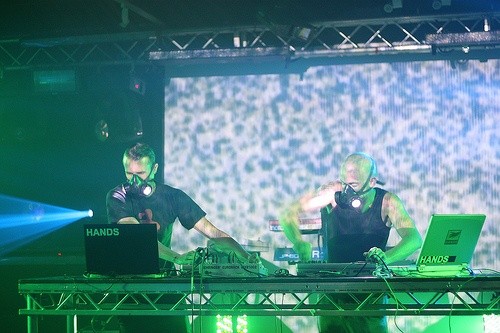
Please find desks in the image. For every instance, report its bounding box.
[17,269,500,333]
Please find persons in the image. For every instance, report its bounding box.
[105,142,261,333]
[278,153,422,333]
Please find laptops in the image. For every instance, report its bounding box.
[84,224,161,277]
[385,213,487,278]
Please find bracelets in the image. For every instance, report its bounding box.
[173,254,180,263]
